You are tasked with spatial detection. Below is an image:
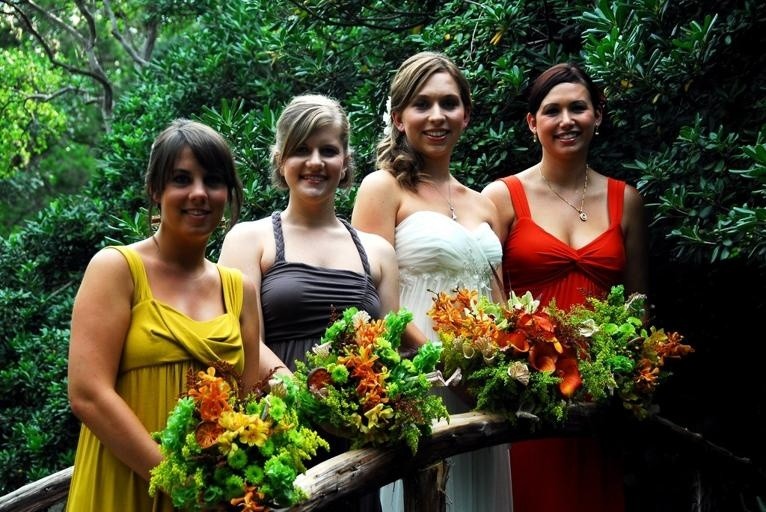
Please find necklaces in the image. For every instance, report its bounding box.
[541,162,590,222]
[429,176,460,220]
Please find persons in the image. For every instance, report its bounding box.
[66,118,260,512]
[481,61,653,511]
[216,93,400,511]
[351,49,515,512]
